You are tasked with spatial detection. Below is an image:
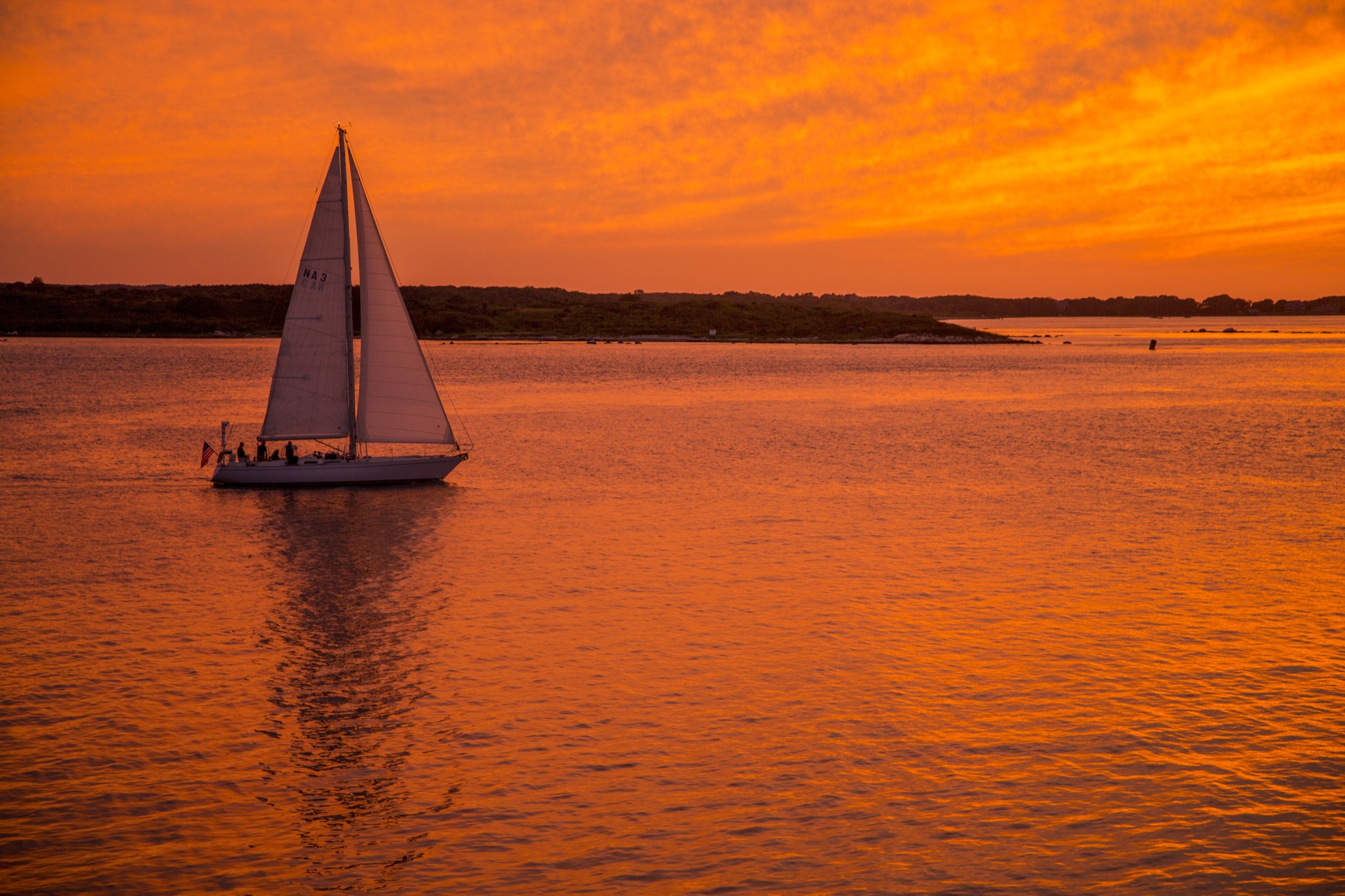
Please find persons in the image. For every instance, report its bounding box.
[285,441,298,460]
[271,450,279,460]
[258,441,267,461]
[237,442,245,459]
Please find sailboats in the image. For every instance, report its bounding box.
[207,119,476,491]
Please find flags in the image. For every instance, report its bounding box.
[201,442,214,468]
